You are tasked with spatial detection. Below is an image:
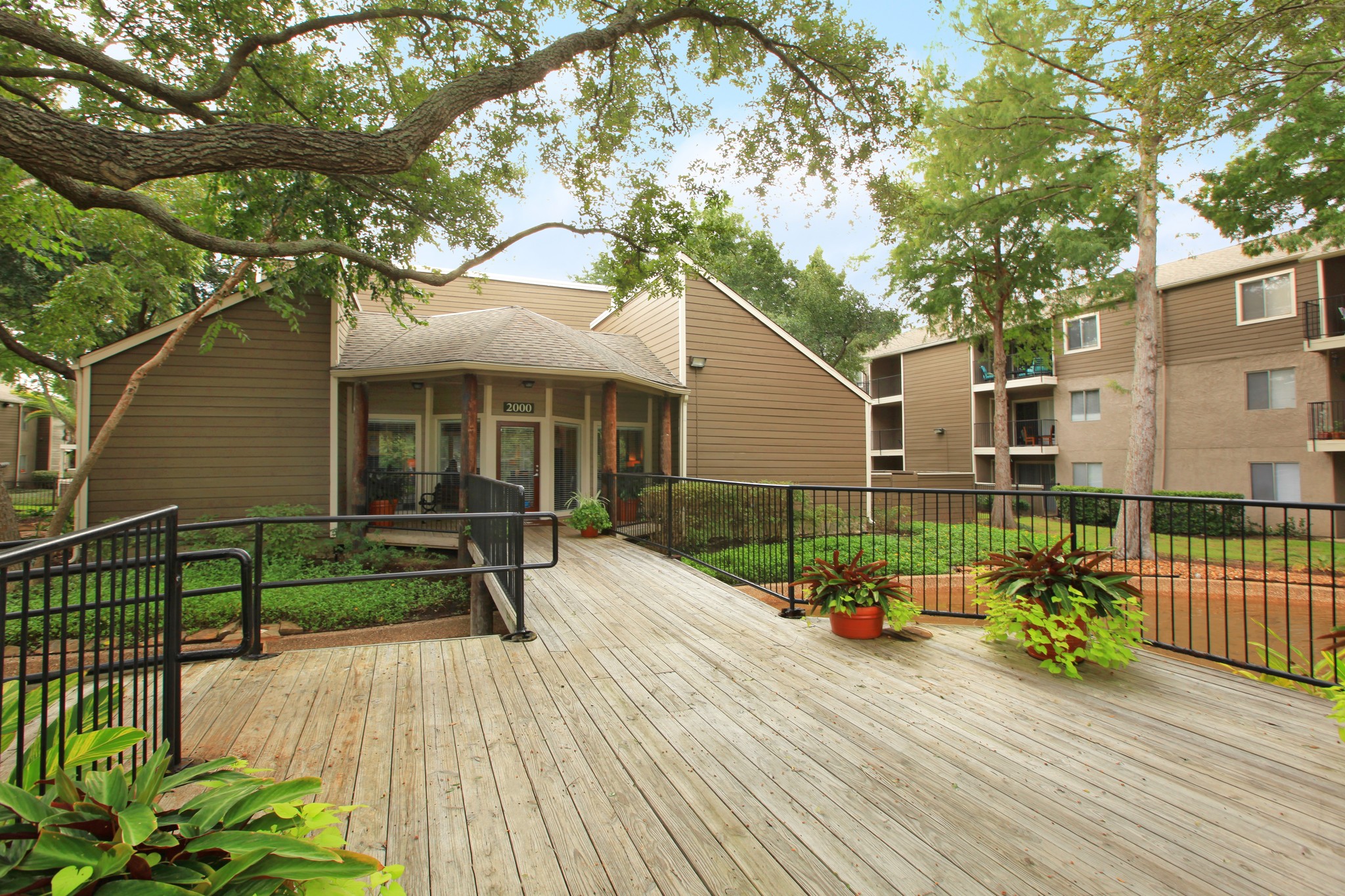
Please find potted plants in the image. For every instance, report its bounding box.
[1330,420,1345,439]
[361,464,404,527]
[1317,410,1333,440]
[610,469,639,521]
[563,488,613,537]
[788,549,924,640]
[964,533,1152,681]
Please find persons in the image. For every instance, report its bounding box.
[445,459,457,473]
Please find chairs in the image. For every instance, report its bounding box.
[1024,427,1041,446]
[980,366,994,381]
[1019,358,1049,377]
[1041,425,1055,445]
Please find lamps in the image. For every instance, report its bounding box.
[522,380,535,388]
[412,382,423,390]
[689,355,706,368]
[933,428,943,434]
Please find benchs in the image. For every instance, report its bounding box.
[419,483,441,524]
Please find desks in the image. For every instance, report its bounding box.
[1006,370,1025,378]
